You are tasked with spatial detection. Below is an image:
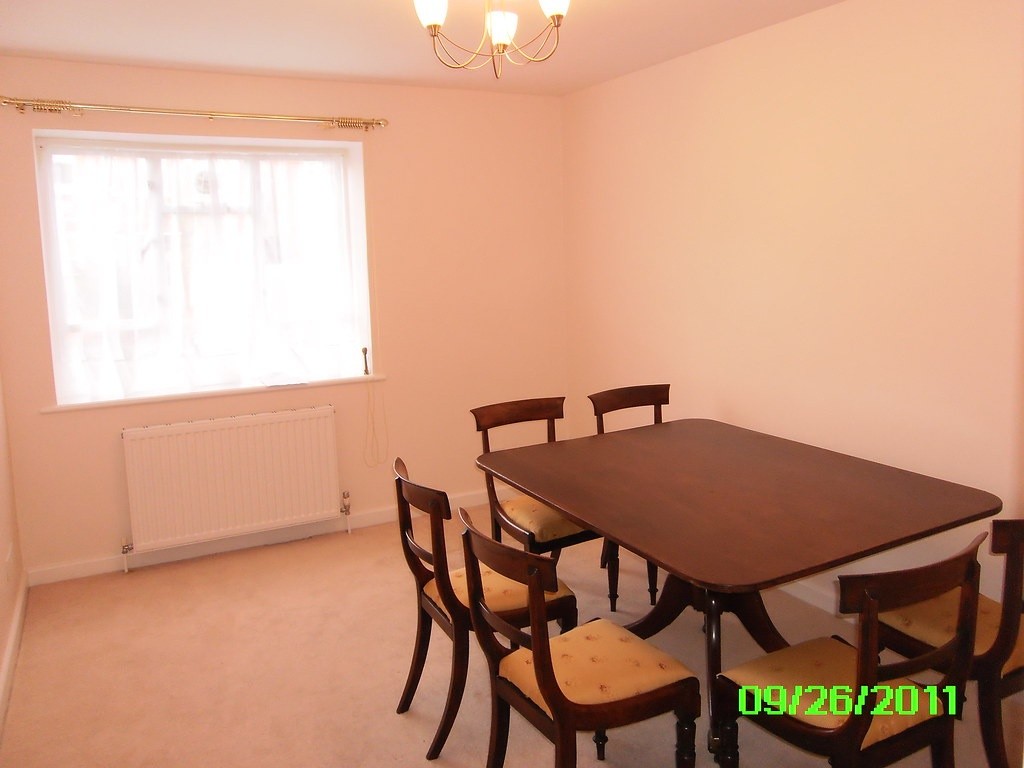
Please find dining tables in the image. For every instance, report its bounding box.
[473,416,1005,755]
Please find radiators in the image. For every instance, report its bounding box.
[121,403,341,555]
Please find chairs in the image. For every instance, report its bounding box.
[713,530,991,768]
[459,505,701,768]
[391,457,580,760]
[469,395,619,613]
[877,518,1024,767]
[587,382,671,606]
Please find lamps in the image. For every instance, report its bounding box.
[413,0,570,80]
